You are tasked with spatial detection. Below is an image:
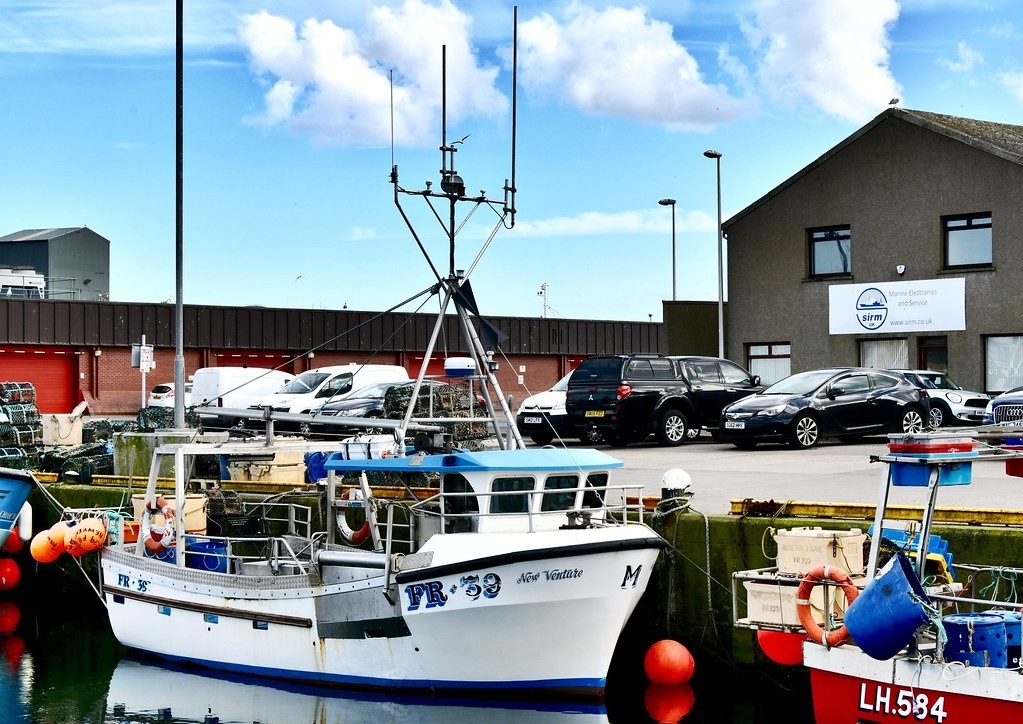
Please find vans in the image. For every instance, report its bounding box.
[191,366,312,432]
[244,363,410,439]
[517,368,605,446]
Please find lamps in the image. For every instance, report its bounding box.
[307,351,316,359]
[93,346,103,358]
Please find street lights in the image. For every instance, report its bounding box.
[657,198,679,301]
[702,149,728,358]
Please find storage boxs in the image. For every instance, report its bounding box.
[743,526,874,627]
[206,514,262,538]
[338,434,398,459]
[886,431,979,487]
[1001,438,1023,477]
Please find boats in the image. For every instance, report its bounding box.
[0,464,39,554]
[59,1,675,696]
[729,434,1023,724]
[101,650,612,724]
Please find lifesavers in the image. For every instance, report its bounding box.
[338,490,374,543]
[141,500,174,553]
[796,566,858,649]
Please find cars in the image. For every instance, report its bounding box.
[147,381,193,415]
[308,379,489,435]
[982,385,1023,432]
[720,367,931,451]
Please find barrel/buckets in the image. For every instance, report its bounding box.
[144,532,229,574]
[844,549,1023,672]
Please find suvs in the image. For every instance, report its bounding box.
[882,367,992,428]
[563,350,772,448]
